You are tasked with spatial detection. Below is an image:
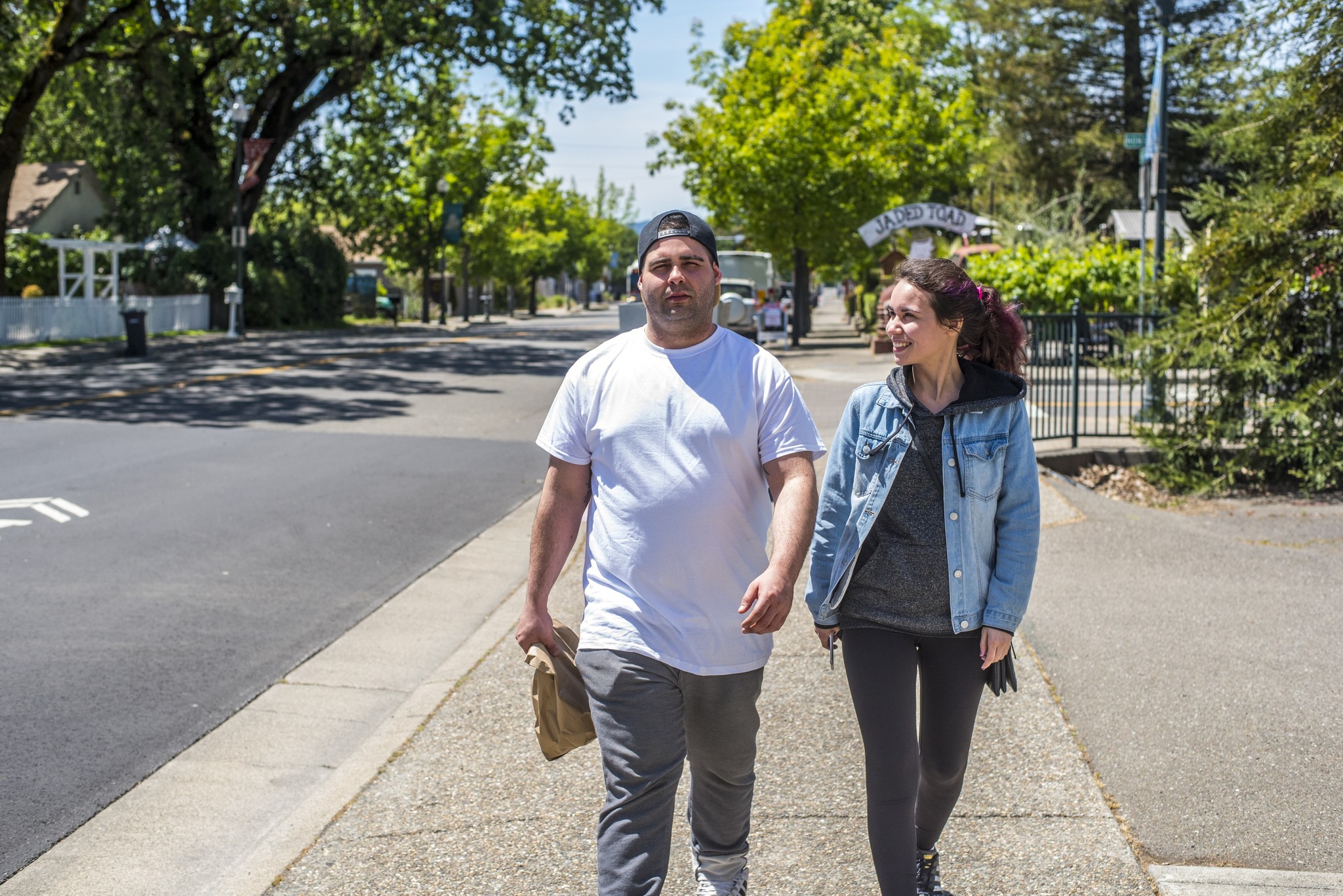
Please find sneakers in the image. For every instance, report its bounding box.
[917,846,943,896]
[693,867,749,896]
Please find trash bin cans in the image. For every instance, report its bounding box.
[119,308,147,355]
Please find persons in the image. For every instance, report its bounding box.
[514,208,831,896]
[803,257,1043,896]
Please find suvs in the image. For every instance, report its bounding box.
[712,276,760,344]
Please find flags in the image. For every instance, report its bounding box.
[238,138,273,192]
[1144,33,1163,158]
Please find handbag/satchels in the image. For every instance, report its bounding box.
[986,644,1017,697]
[526,617,598,761]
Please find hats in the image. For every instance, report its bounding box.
[637,210,721,307]
